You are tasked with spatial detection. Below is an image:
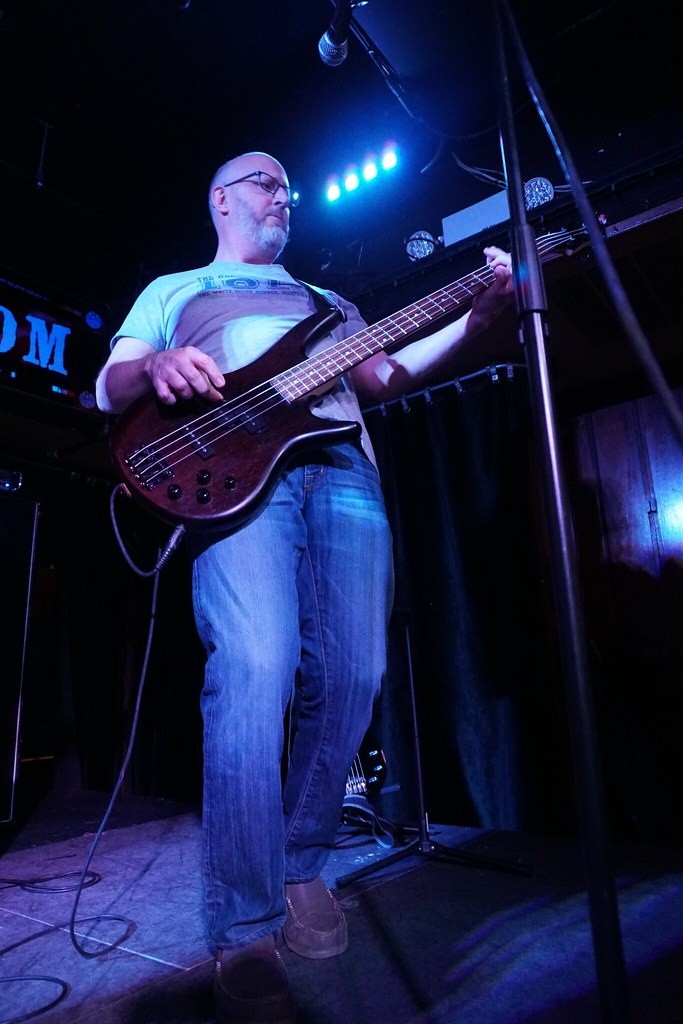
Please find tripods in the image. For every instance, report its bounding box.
[334,604,537,890]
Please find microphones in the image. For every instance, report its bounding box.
[317,0,353,67]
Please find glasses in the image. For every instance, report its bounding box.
[212,170,305,209]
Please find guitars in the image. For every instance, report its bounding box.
[343,734,387,804]
[112,211,609,532]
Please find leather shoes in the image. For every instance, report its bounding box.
[282,874,348,958]
[213,934,297,1024]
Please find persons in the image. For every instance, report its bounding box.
[95,152,512,1024]
